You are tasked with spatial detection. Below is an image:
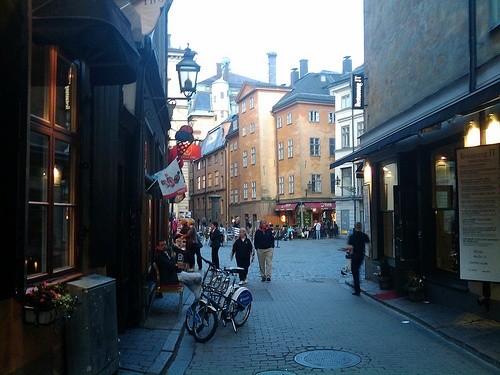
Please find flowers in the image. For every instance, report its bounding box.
[29,279,69,310]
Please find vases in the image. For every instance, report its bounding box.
[23,303,72,328]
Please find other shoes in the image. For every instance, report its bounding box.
[209,267,221,272]
[262,278,266,281]
[239,281,244,285]
[244,281,247,284]
[267,278,271,281]
[352,290,360,296]
[188,268,195,272]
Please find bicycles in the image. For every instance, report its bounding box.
[184,255,253,343]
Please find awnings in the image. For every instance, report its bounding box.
[32,0,141,86]
[305,202,336,210]
[275,203,298,211]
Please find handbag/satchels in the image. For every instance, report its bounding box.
[193,241,203,248]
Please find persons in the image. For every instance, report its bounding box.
[171,217,202,272]
[271,220,338,241]
[209,221,221,271]
[246,217,252,230]
[230,228,253,285]
[346,222,372,296]
[253,219,275,282]
[153,238,202,300]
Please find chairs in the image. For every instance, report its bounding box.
[146,262,185,322]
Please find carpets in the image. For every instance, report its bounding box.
[372,292,402,301]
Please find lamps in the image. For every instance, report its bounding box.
[143,46,202,126]
